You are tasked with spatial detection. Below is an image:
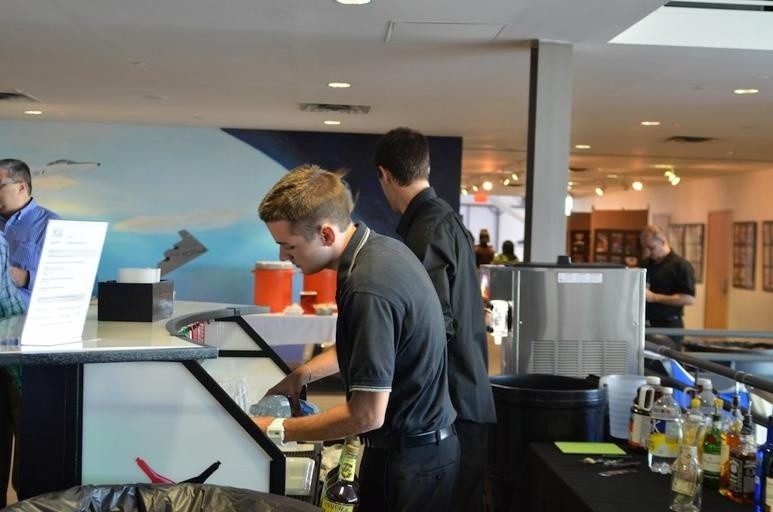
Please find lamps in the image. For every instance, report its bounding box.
[461,166,681,196]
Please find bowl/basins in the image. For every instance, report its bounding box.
[490,371,608,445]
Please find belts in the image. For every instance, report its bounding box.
[358,422,457,450]
[649,315,681,322]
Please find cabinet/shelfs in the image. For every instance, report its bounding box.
[566,211,590,264]
[587,203,652,263]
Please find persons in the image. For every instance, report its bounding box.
[251,163,460,512]
[375,127,497,511]
[474,230,494,268]
[639,225,695,345]
[495,240,517,265]
[0,159,61,318]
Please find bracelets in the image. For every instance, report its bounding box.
[304,364,311,383]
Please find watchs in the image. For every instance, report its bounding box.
[266,417,285,447]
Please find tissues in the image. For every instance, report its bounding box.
[99,267,174,323]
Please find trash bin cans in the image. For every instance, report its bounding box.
[1,481,334,512]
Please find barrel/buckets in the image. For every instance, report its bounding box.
[486,375,606,512]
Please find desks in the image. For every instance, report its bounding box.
[529,440,773,511]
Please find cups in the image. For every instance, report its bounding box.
[232,378,250,416]
[311,303,333,315]
[299,291,317,315]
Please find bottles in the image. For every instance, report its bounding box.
[135,457,177,485]
[249,393,317,419]
[318,433,365,512]
[179,461,222,485]
[623,375,773,512]
